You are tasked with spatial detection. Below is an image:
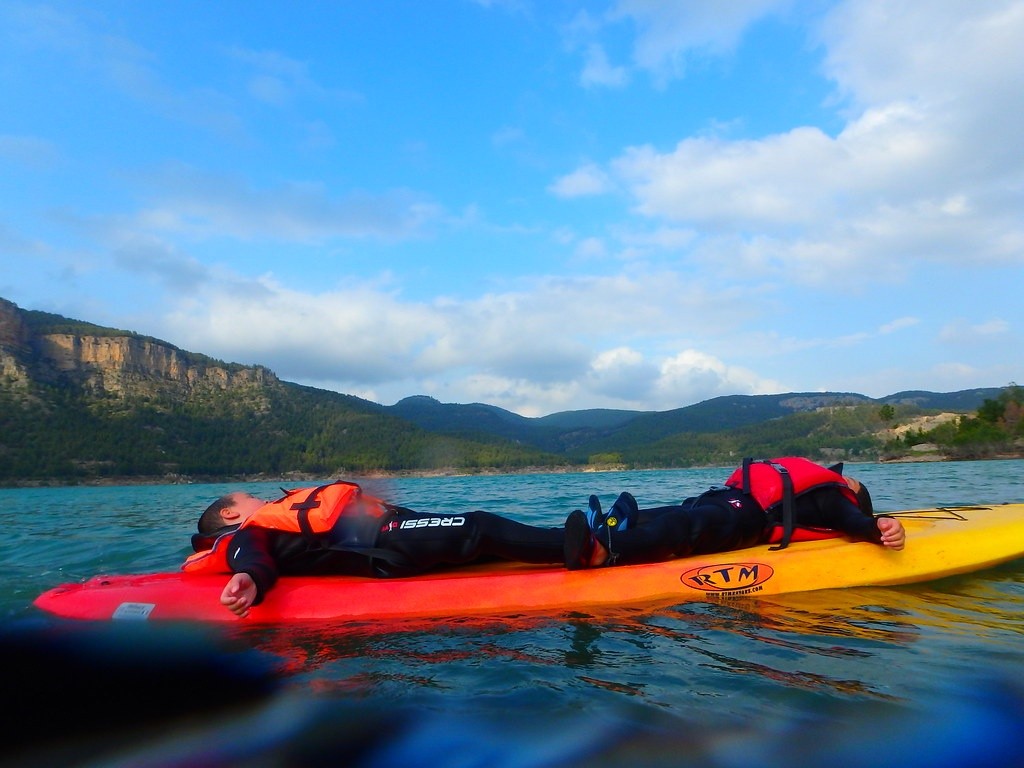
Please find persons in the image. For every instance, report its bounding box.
[192,483,592,619]
[588,458,907,564]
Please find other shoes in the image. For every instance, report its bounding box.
[596,491,639,539]
[563,510,594,571]
[584,495,603,532]
